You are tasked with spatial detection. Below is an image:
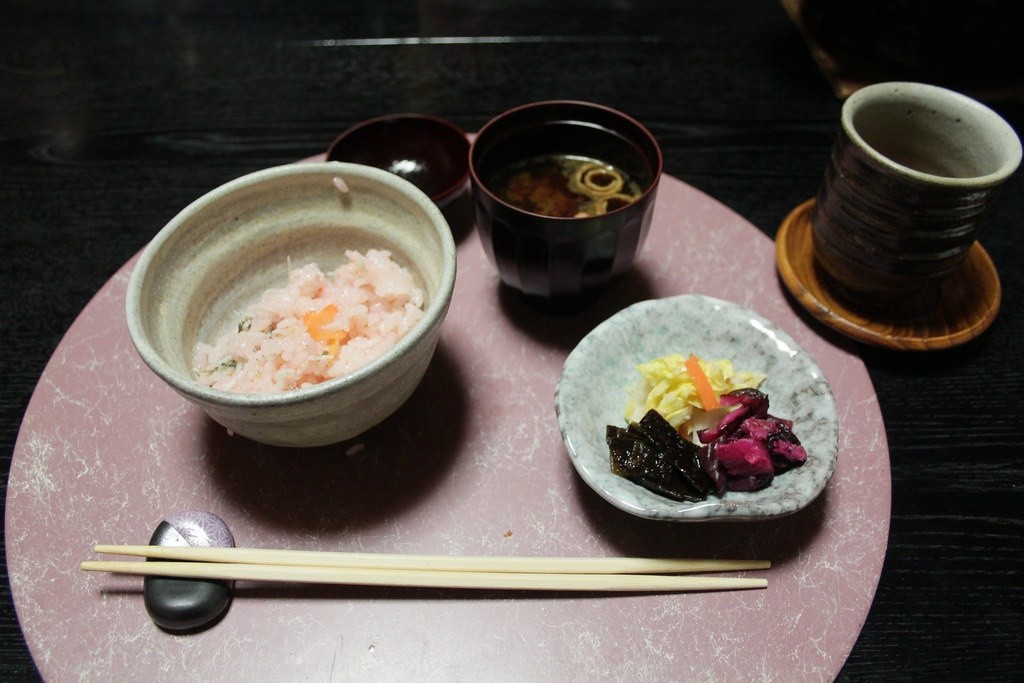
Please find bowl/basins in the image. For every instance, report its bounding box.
[125,161,456,446]
[468,99,662,299]
[326,113,473,244]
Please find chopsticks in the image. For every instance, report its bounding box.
[80,545,772,591]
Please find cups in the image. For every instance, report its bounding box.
[810,81,1023,316]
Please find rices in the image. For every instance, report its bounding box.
[193,178,425,395]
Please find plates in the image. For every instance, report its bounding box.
[553,294,838,522]
[773,197,1002,350]
